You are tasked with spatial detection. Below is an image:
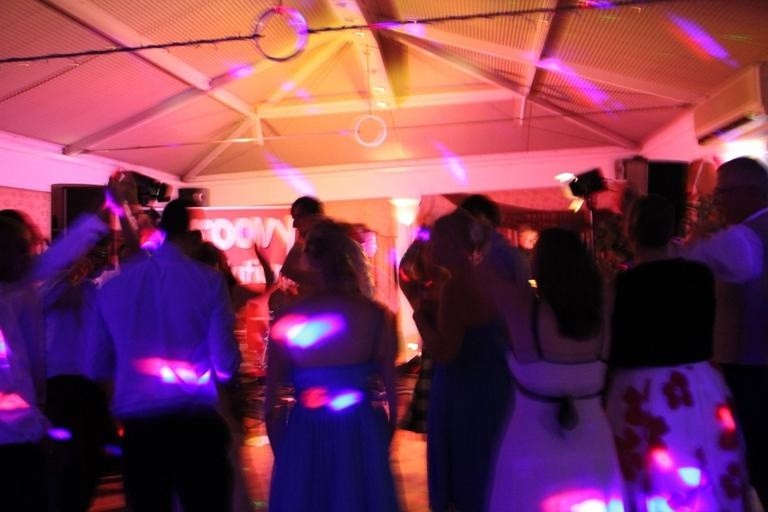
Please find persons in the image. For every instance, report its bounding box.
[663,157,766,510]
[467,212,629,511]
[400,194,532,509]
[264,218,406,510]
[604,196,763,511]
[268,196,335,326]
[2,194,123,510]
[76,169,251,510]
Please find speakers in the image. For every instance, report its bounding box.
[51,183,116,271]
[623,159,688,237]
